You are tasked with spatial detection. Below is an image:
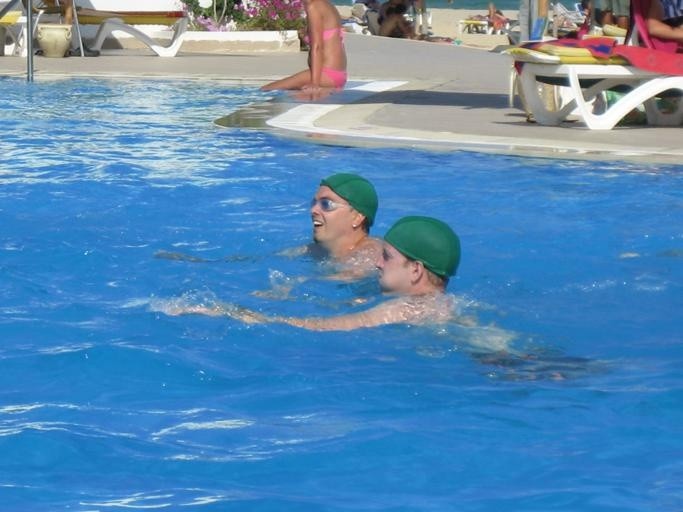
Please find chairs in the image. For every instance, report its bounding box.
[458,19,519,35]
[0,1,91,57]
[509,0,683,130]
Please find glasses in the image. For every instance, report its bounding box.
[309,197,350,212]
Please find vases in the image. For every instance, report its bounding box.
[35,22,72,56]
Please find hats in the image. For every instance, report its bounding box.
[383,214,461,279]
[319,171,379,226]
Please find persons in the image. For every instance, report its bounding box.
[173,213,603,386]
[149,172,386,283]
[598,0,628,29]
[642,1,682,53]
[488,3,495,18]
[581,0,590,17]
[364,1,426,40]
[494,11,505,33]
[258,0,346,95]
